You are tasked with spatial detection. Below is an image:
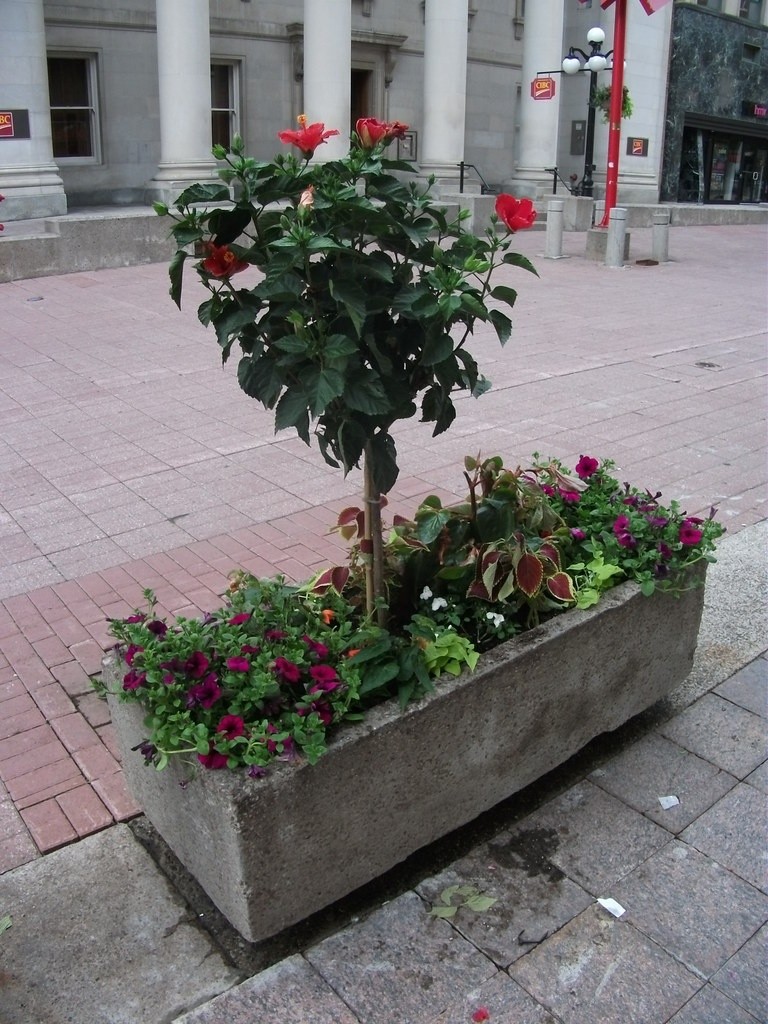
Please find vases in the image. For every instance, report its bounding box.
[99,552,706,944]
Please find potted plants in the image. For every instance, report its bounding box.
[586,82,634,124]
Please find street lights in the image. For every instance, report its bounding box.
[561,26,627,197]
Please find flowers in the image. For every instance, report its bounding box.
[106,115,729,779]
